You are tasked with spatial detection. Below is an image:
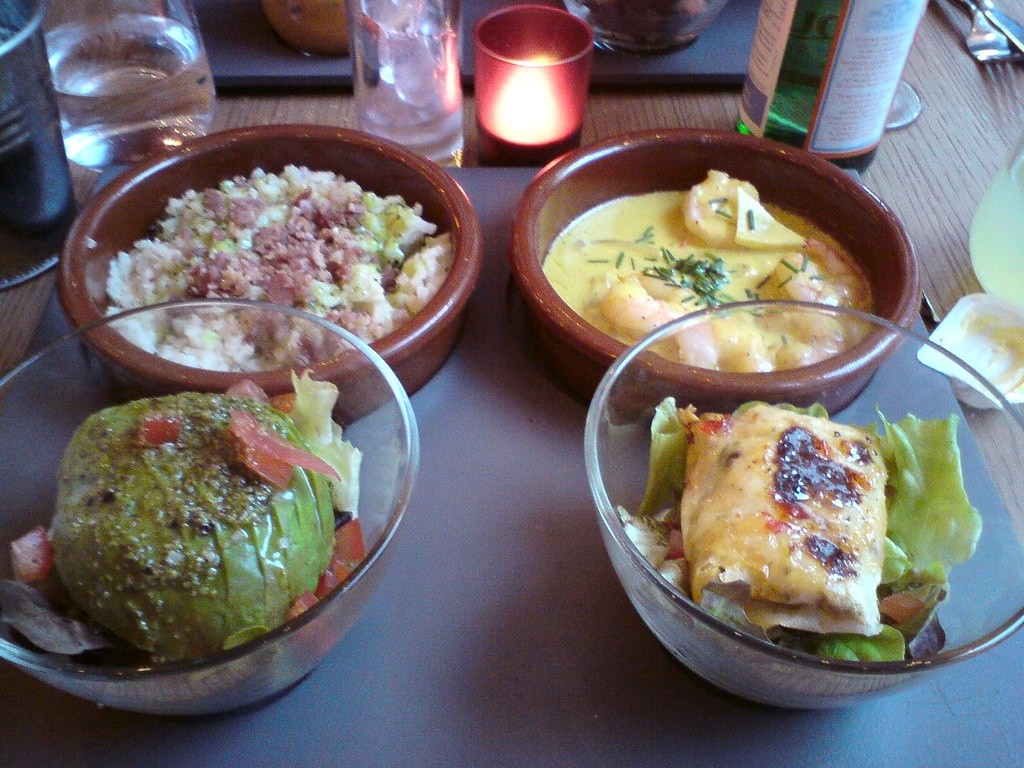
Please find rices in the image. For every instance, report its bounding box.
[104,165,454,372]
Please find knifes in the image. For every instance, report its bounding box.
[973,0,1024,53]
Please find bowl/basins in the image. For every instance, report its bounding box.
[58,121,485,427]
[509,125,921,423]
[583,298,1024,710]
[0,300,422,720]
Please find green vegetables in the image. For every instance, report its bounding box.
[636,396,983,662]
[586,198,824,318]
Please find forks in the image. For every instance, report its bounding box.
[949,0,1024,64]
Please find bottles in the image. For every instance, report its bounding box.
[739,0,932,177]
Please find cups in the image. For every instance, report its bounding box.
[346,1,468,170]
[0,0,80,289]
[564,0,730,61]
[40,0,218,171]
[471,4,595,167]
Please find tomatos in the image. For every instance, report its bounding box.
[8,379,366,660]
